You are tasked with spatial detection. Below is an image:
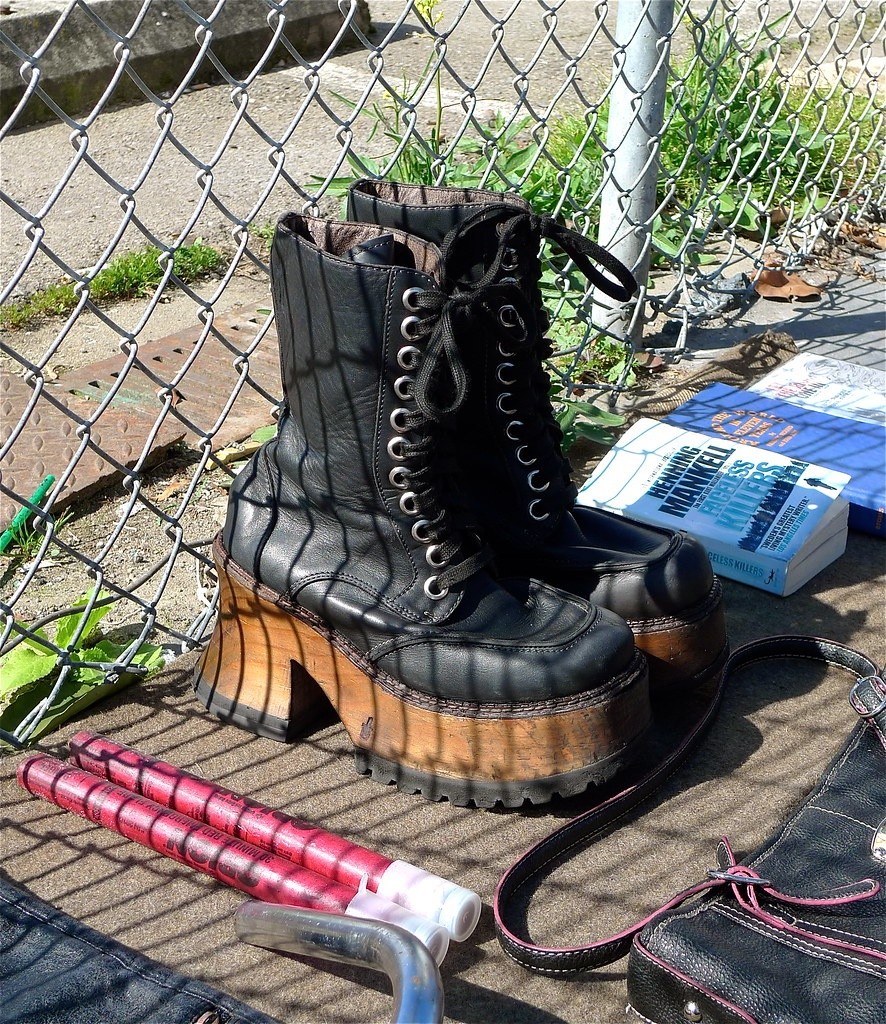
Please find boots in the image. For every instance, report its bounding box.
[193,211,652,808]
[346,178,730,708]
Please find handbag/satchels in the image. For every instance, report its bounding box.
[493,632,886,1024]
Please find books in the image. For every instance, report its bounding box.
[576,352,886,596]
